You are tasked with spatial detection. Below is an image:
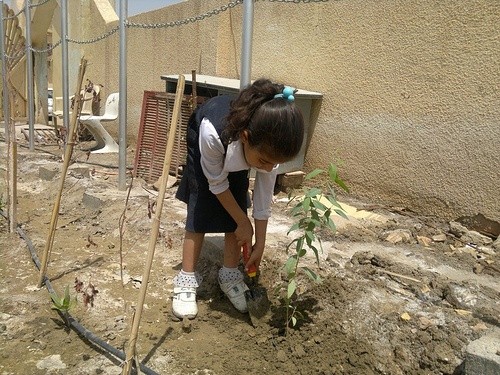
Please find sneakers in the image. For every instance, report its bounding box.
[172,287,198,319]
[219,274,250,312]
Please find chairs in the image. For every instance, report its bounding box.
[53,83,120,153]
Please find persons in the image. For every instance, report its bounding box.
[171,79,304,319]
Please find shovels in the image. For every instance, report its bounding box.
[241,238,271,329]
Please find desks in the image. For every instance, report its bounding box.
[160,72,323,176]
